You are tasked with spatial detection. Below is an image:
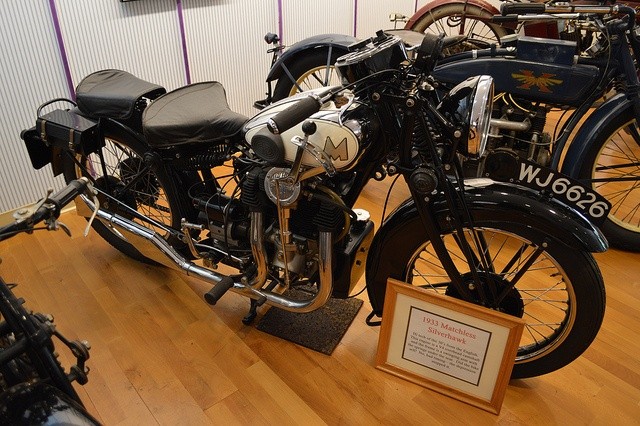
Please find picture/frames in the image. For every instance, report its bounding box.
[373,276,526,415]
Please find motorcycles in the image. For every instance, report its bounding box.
[403,0,639,60]
[0,176,101,426]
[20,29,611,380]
[255,3,640,252]
